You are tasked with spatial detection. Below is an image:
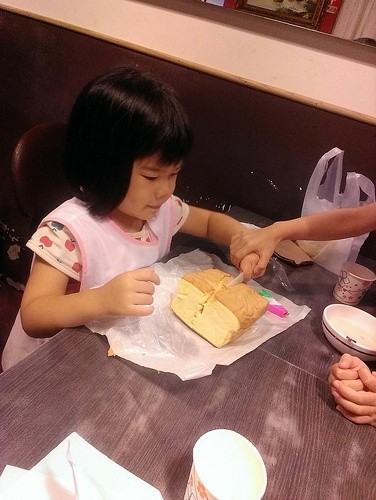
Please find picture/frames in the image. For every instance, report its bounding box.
[234,0,329,31]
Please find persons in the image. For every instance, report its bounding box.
[229,201,376,274]
[1,67,265,372]
[328,353,376,427]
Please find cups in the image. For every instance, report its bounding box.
[183,429,267,500]
[333,262,376,306]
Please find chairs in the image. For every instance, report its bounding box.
[13,122,78,269]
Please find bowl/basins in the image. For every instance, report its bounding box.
[322,304,376,362]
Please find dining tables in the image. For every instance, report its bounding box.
[0,205,376,500]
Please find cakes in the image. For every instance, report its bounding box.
[171,269,269,348]
